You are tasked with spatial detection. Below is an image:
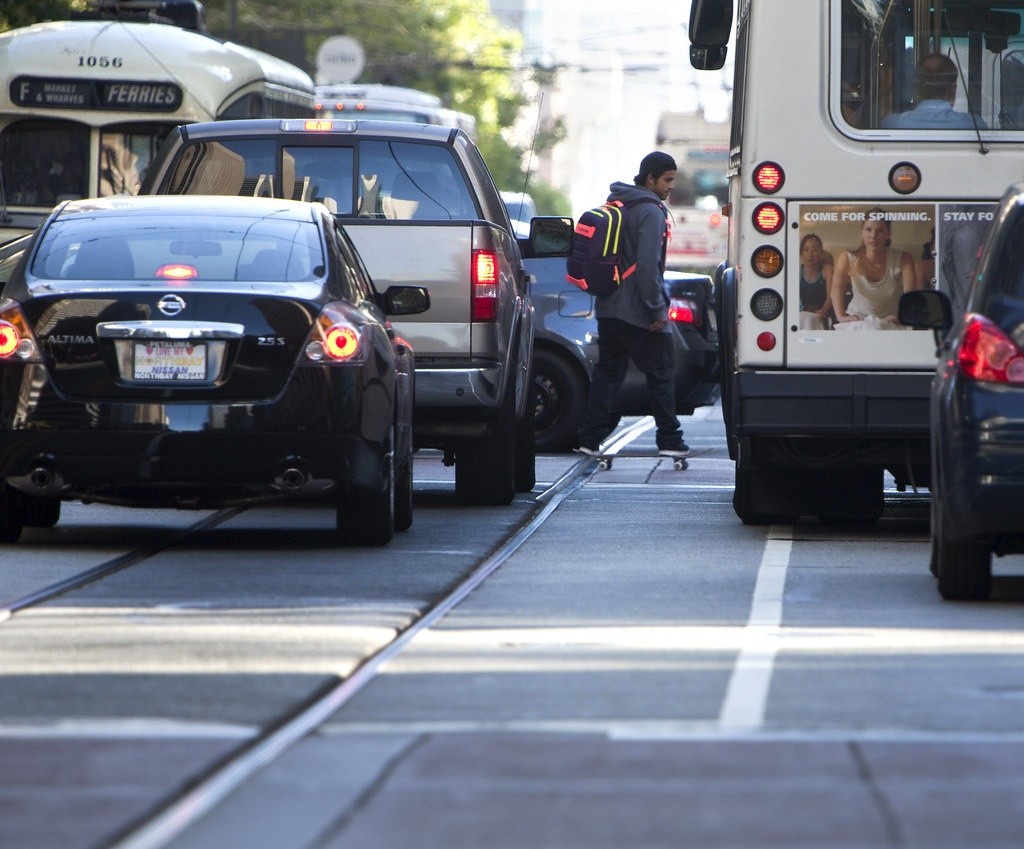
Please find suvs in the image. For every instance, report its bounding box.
[134,116,576,505]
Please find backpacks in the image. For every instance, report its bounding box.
[565,200,672,298]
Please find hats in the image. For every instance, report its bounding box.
[634,152,682,184]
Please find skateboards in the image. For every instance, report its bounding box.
[568,445,719,474]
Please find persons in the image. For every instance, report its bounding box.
[799,233,834,330]
[573,151,691,459]
[830,207,916,331]
[877,52,988,130]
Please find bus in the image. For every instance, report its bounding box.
[315,84,479,229]
[684,0,1024,531]
[1,20,316,240]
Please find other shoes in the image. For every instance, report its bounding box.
[579,437,600,456]
[655,429,694,455]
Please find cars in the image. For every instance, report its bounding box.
[511,211,725,459]
[896,184,1024,598]
[1,196,432,549]
